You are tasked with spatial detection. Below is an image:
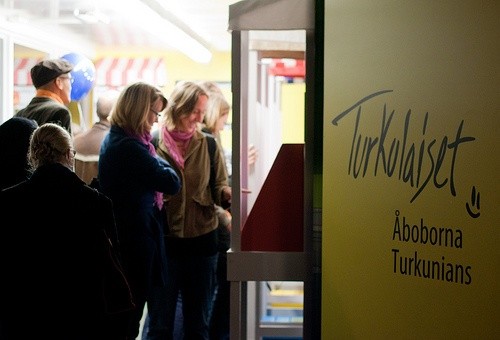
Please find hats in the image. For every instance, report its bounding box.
[30,59,73,89]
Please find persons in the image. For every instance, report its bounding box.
[0,57,118,189]
[95,84,182,340]
[142,81,232,340]
[0,122,119,340]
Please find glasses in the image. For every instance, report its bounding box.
[149,108,161,118]
[59,77,74,83]
